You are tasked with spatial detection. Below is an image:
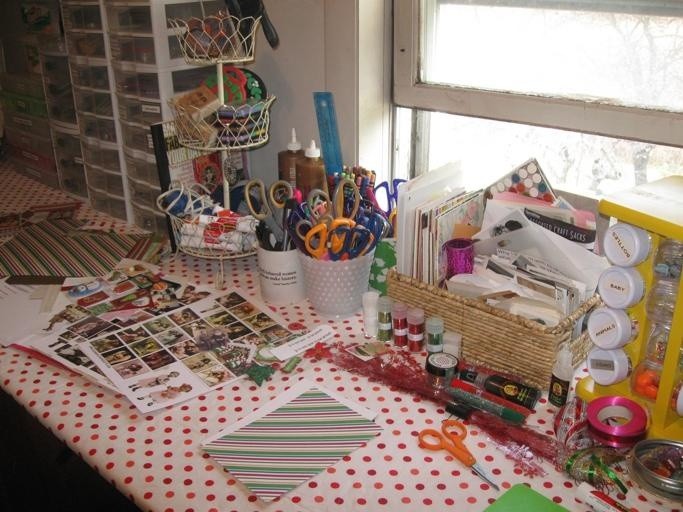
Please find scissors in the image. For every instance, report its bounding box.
[246,174,411,261]
[418,419,501,490]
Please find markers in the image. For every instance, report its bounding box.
[443,385,524,422]
[450,377,532,417]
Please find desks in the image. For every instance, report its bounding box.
[0,248,683,512]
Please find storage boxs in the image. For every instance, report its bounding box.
[6,141,54,170]
[2,91,48,118]
[4,125,53,159]
[7,156,57,183]
[38,48,79,131]
[48,123,91,205]
[3,109,51,138]
[1,71,47,98]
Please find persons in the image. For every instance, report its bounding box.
[120,329,145,341]
[231,302,256,318]
[202,371,229,385]
[179,285,211,305]
[170,311,192,326]
[131,341,156,356]
[118,363,141,377]
[43,304,73,331]
[129,370,179,392]
[217,293,241,307]
[75,319,104,334]
[198,328,229,350]
[137,384,192,407]
[159,330,182,345]
[224,323,245,337]
[187,357,211,370]
[171,339,201,358]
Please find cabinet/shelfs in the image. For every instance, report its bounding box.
[100,1,250,235]
[59,0,133,227]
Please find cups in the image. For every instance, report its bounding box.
[255,245,306,308]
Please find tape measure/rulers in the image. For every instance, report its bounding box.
[311,90,343,200]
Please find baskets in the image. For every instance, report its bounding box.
[386,265,601,390]
